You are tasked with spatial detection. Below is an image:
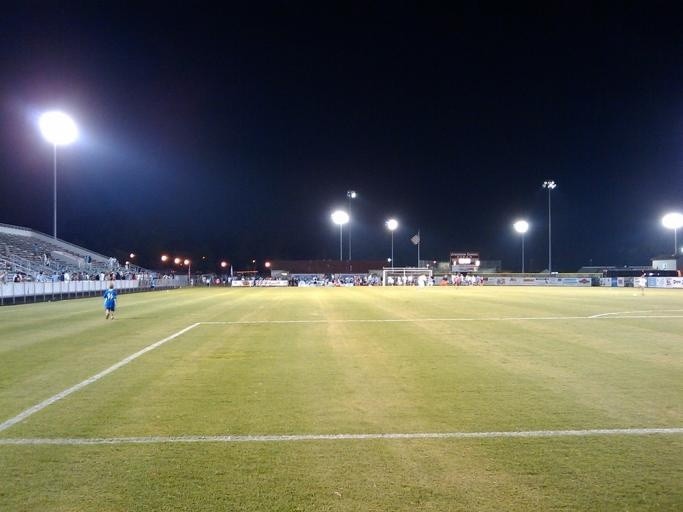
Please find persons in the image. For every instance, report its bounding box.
[212,272,486,287]
[0,240,211,291]
[636,273,647,296]
[102,282,118,320]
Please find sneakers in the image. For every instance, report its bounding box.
[105,313,114,320]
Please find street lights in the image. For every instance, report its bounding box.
[334,190,357,262]
[542,180,556,272]
[517,220,529,273]
[669,213,681,257]
[42,110,68,235]
[388,219,398,268]
